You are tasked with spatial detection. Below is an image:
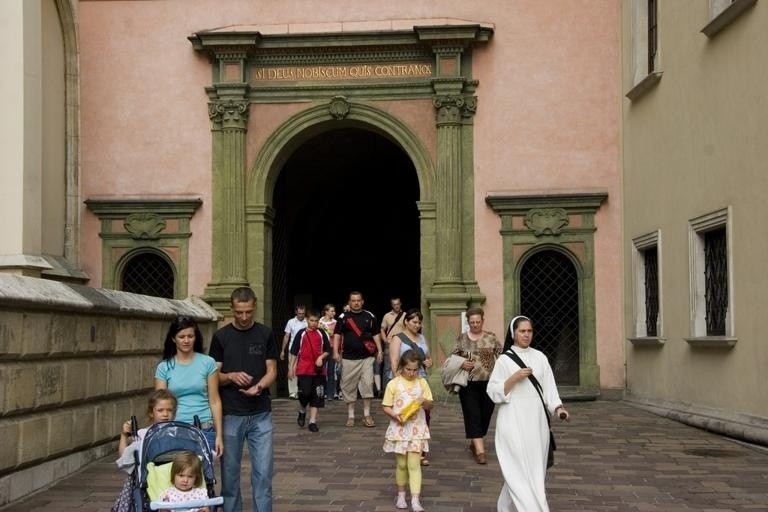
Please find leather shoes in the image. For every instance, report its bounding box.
[298,410,306,427]
[473,444,486,464]
[308,423,318,432]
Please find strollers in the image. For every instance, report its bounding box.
[110,414,224,511]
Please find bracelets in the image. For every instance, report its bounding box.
[255,389,262,396]
[554,405,564,412]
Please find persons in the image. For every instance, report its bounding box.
[381,348,434,511]
[446,307,506,466]
[157,450,212,512]
[485,315,572,511]
[208,286,278,512]
[153,315,224,512]
[280,293,433,467]
[111,388,178,512]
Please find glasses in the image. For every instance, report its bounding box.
[177,315,194,322]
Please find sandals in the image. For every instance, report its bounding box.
[420,457,429,467]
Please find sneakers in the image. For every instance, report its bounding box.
[394,492,409,509]
[346,417,356,427]
[411,497,424,512]
[289,393,296,400]
[362,415,375,426]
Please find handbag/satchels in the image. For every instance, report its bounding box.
[362,333,378,355]
[547,432,556,469]
